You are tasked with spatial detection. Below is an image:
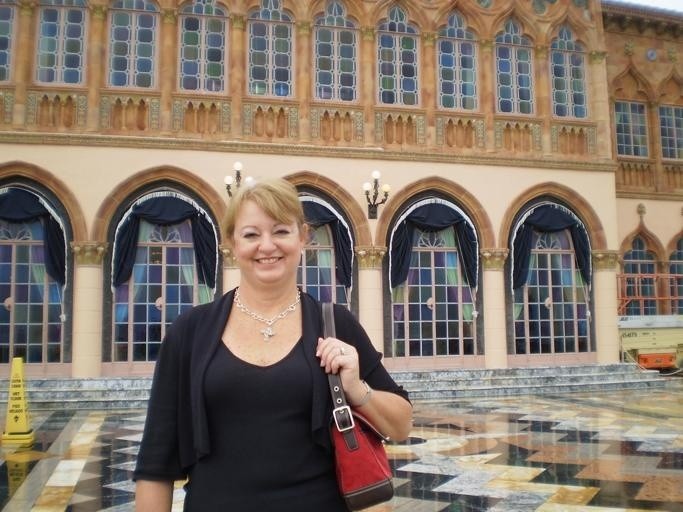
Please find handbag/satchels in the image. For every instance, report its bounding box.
[319,301,395,511]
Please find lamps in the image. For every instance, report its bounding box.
[222,162,254,201]
[360,168,390,220]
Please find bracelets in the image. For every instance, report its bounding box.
[346,379,373,408]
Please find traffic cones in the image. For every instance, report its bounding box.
[0,440,33,502]
[0,352,36,441]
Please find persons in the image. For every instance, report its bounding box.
[129,175,417,512]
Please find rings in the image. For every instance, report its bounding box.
[339,345,345,355]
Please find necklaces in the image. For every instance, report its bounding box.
[231,285,302,342]
